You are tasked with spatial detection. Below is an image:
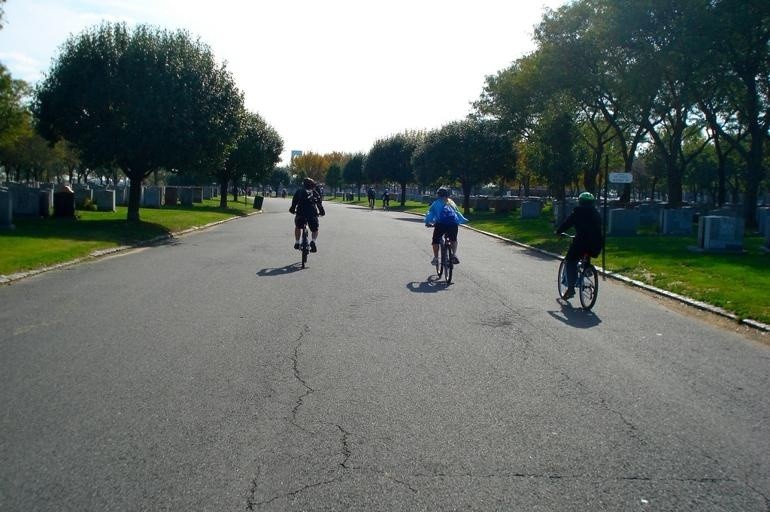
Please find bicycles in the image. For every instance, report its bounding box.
[291,208,324,268]
[369,197,375,210]
[554,229,599,311]
[381,196,390,212]
[425,223,460,285]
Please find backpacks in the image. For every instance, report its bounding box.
[296,188,317,218]
[436,198,459,226]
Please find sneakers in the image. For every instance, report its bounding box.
[453,257,460,264]
[294,244,299,249]
[431,259,438,265]
[310,240,316,253]
[562,289,575,300]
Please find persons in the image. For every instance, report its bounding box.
[553,191,603,300]
[380,189,390,210]
[366,187,376,207]
[423,187,470,265]
[262,191,272,198]
[345,191,354,201]
[289,177,325,252]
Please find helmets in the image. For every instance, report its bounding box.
[305,177,316,188]
[436,187,451,197]
[577,191,595,204]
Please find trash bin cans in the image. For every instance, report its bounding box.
[253,196,263,210]
